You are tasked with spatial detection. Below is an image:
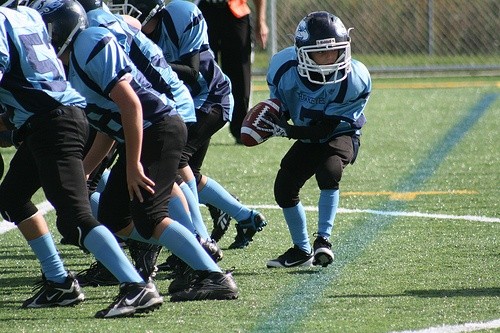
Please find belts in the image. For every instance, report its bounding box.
[16,109,62,142]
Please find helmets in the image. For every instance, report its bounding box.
[107,0,165,28]
[29,0,89,58]
[78,0,102,13]
[294,11,353,84]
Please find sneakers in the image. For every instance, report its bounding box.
[227,211,267,249]
[75,261,120,287]
[22,269,85,308]
[158,230,239,301]
[205,193,242,243]
[124,239,163,282]
[266,245,314,268]
[313,232,334,267]
[94,282,163,319]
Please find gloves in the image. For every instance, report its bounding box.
[257,110,298,141]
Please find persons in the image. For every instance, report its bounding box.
[257,11,372,268]
[0,0,270,319]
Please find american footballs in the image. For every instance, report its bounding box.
[240,98,282,146]
